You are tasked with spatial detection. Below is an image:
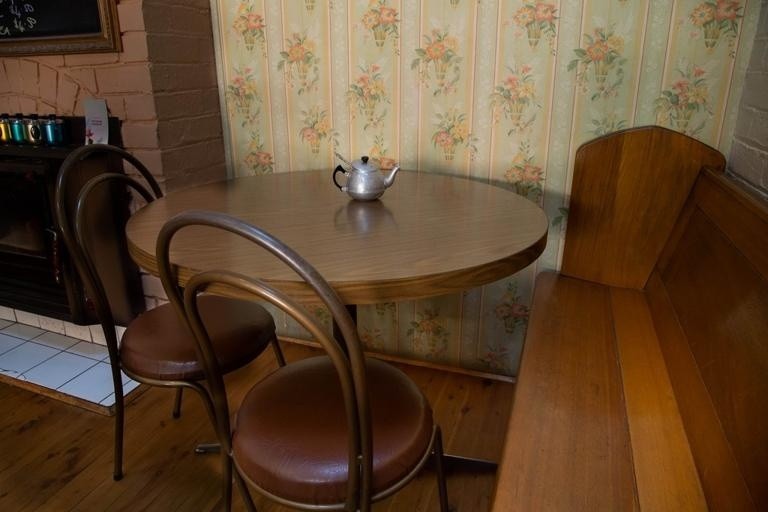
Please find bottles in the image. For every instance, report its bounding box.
[0,112,67,144]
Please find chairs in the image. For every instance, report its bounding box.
[54,144,286,511]
[156,210,449,511]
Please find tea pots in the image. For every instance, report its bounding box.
[331,154,403,203]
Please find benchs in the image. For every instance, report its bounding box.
[490,123,766,512]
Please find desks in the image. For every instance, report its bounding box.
[117,164,547,482]
